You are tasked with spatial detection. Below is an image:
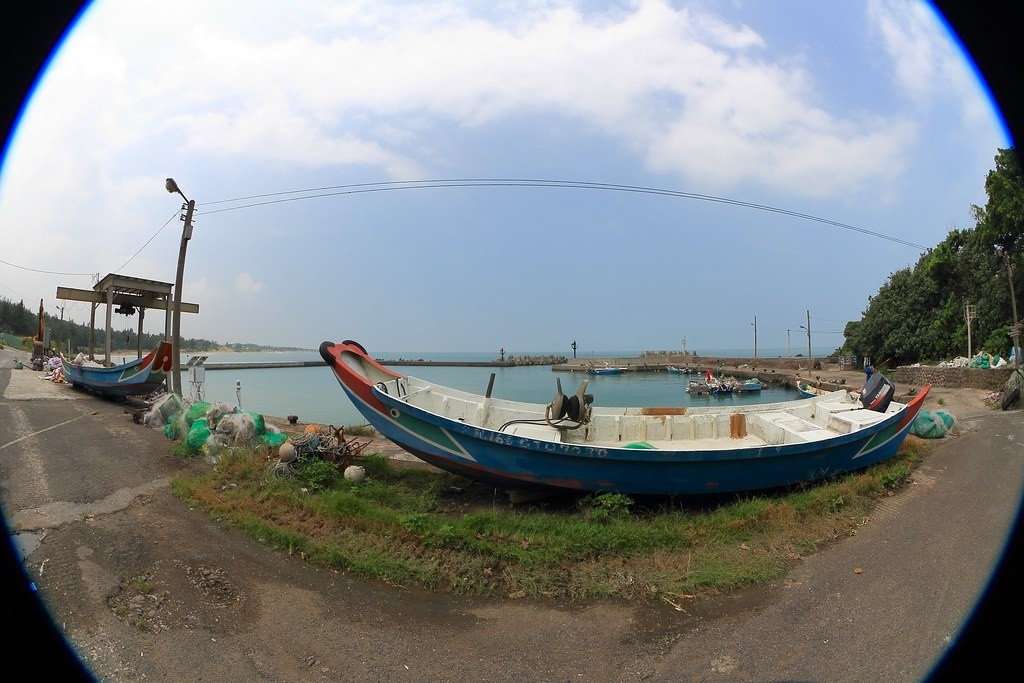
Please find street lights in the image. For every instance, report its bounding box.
[992,243,1020,397]
[800,309,812,376]
[165,178,197,404]
[750,315,757,359]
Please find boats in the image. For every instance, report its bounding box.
[665,365,693,374]
[585,362,628,375]
[59,340,172,398]
[686,378,763,396]
[318,338,931,501]
[795,380,832,398]
